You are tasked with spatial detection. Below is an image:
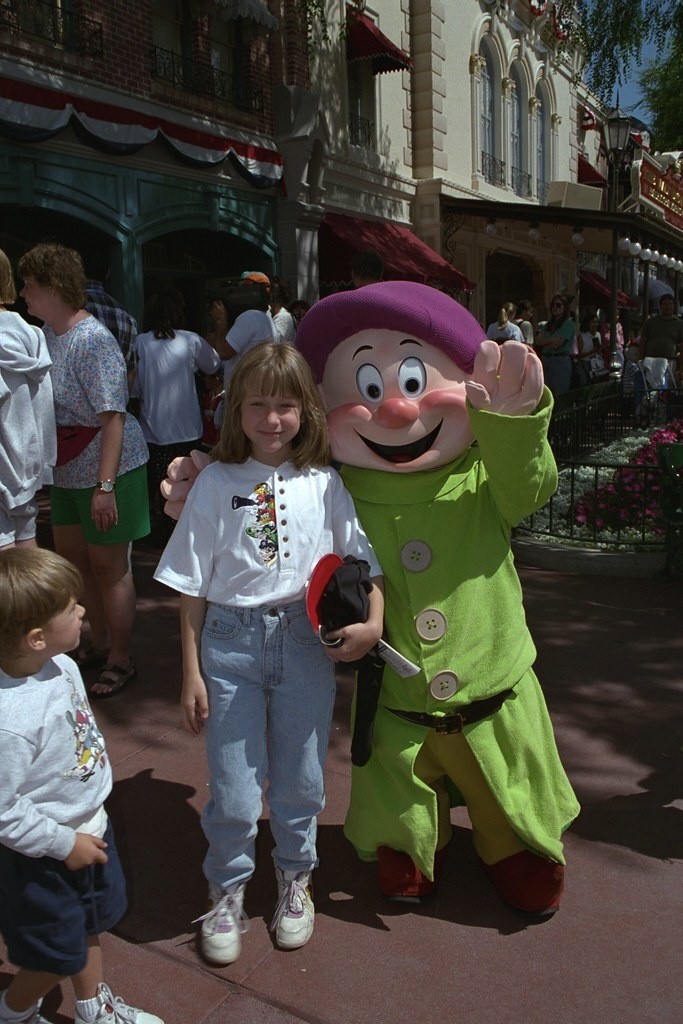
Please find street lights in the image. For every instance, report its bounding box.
[600,88,634,384]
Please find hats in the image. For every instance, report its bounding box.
[304,553,369,646]
[238,271,271,292]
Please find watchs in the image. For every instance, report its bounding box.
[97,479,115,493]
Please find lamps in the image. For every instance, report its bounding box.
[528,220,540,239]
[617,229,683,273]
[485,216,497,235]
[571,224,585,245]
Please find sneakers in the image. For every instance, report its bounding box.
[190,878,250,963]
[270,868,314,947]
[0,993,53,1024]
[72,981,163,1024]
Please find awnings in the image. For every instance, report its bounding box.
[579,153,609,187]
[580,269,638,310]
[216,0,279,32]
[318,213,476,295]
[346,2,414,77]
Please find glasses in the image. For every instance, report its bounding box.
[550,302,565,309]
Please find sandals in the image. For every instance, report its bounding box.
[70,649,108,669]
[89,656,136,697]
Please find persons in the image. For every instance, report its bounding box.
[0,245,683,1024]
